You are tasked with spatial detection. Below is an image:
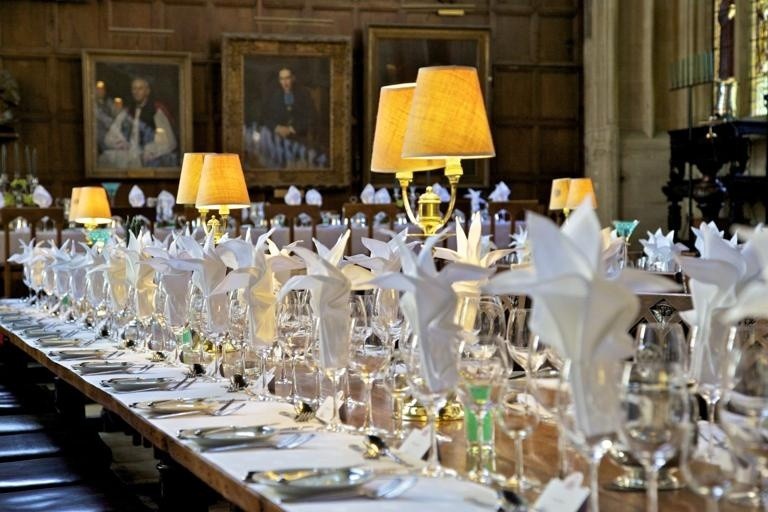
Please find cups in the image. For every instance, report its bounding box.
[634,321,687,386]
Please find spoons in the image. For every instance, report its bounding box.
[112,376,189,393]
[201,432,300,454]
[150,397,236,419]
[281,474,402,503]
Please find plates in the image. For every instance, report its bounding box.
[73,360,134,371]
[254,464,377,492]
[56,350,105,358]
[0,308,43,347]
[105,376,174,391]
[178,425,280,439]
[43,323,78,347]
[129,398,221,414]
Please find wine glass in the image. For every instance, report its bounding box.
[405,331,457,480]
[497,377,546,489]
[382,364,411,420]
[636,256,677,275]
[83,271,104,323]
[319,209,364,228]
[21,258,33,300]
[459,290,506,359]
[281,302,311,405]
[507,304,549,410]
[346,295,371,351]
[311,309,349,431]
[682,422,736,510]
[247,295,279,400]
[460,337,514,486]
[373,286,405,355]
[556,360,629,511]
[41,264,55,310]
[67,269,84,320]
[352,316,393,438]
[52,267,70,317]
[595,235,630,286]
[187,294,209,368]
[157,273,190,359]
[723,294,768,507]
[617,358,687,511]
[107,273,134,341]
[128,276,152,351]
[292,289,308,337]
[200,293,230,383]
[228,288,250,388]
[694,324,738,454]
[32,261,46,307]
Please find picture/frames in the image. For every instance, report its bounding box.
[81,47,193,181]
[362,20,492,190]
[220,30,355,189]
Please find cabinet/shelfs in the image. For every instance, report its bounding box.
[660,121,768,253]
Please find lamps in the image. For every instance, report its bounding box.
[370,63,497,420]
[548,176,599,223]
[176,152,254,355]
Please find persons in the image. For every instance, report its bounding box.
[264,64,321,142]
[104,75,177,168]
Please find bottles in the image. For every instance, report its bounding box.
[465,385,496,475]
